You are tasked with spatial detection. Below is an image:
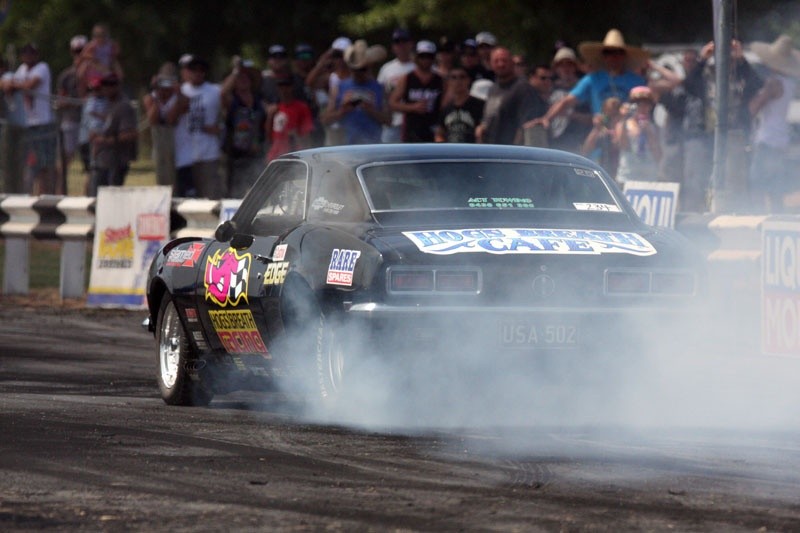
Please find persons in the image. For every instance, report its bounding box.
[2,10,799,224]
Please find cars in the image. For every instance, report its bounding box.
[142,141,706,412]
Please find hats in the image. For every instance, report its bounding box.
[13,27,800,102]
